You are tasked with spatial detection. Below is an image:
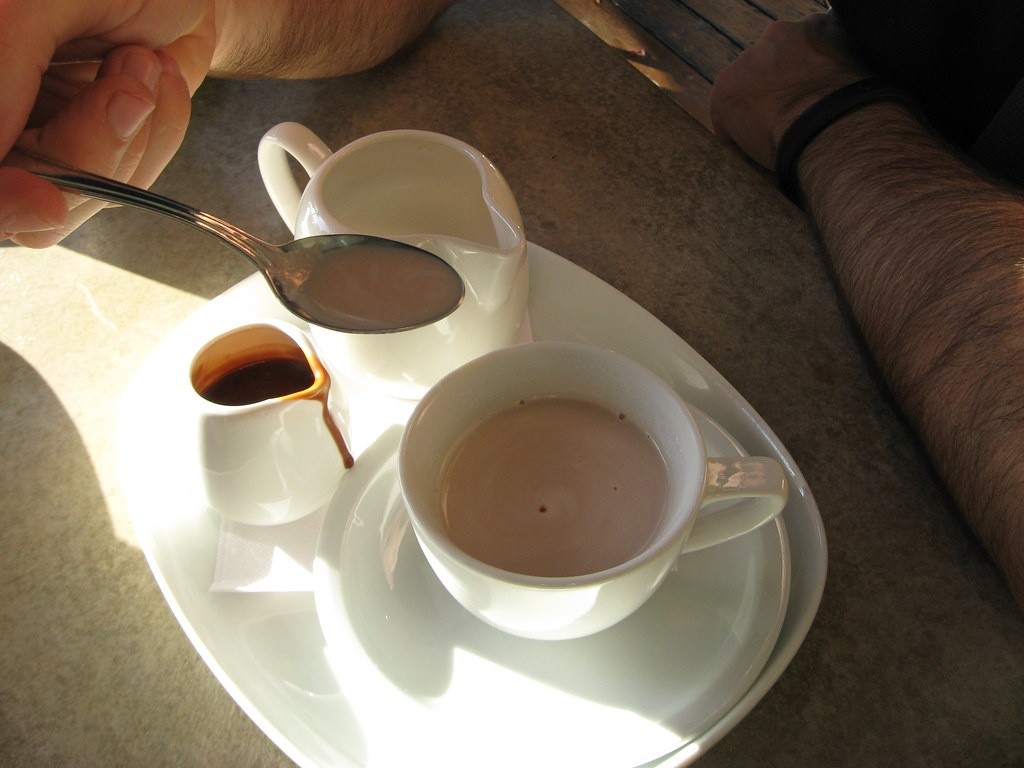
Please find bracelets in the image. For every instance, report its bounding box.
[776,78,930,211]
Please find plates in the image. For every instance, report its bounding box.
[312,398,791,768]
[134,240,827,768]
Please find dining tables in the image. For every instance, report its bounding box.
[0,0,1024,767]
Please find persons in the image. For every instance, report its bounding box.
[1,0,1024,608]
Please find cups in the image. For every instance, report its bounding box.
[179,319,352,529]
[398,343,788,642]
[257,122,530,401]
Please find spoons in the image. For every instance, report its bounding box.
[1,148,466,334]
